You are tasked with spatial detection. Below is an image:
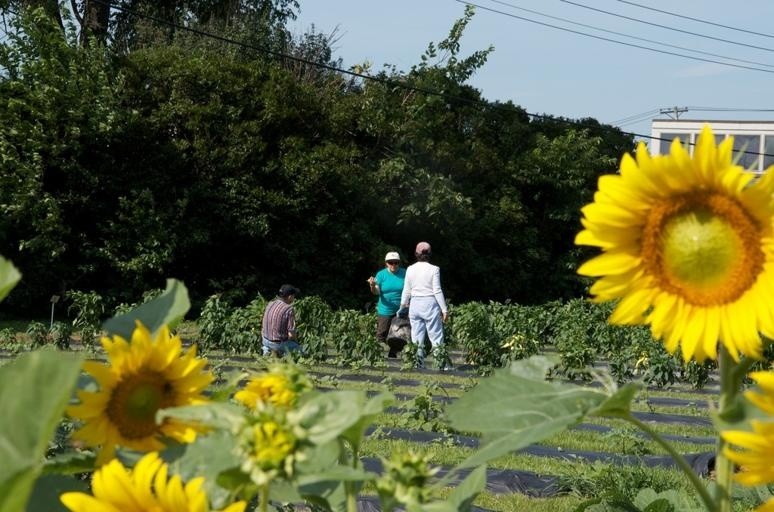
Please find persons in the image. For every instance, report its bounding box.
[400,242,458,371]
[262,285,304,364]
[367,251,410,359]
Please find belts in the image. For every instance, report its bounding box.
[263,336,281,342]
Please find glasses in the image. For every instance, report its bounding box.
[387,260,400,265]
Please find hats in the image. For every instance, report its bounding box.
[416,241,432,255]
[278,284,301,298]
[384,251,400,263]
